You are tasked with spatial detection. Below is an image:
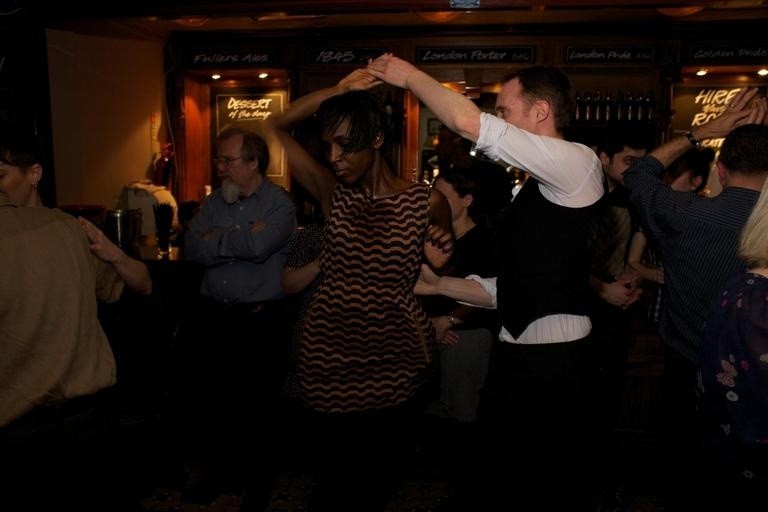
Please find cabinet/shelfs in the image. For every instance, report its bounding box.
[403,64,662,186]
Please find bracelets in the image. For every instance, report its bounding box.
[685,131,697,146]
[446,313,456,328]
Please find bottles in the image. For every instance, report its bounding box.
[573,89,656,125]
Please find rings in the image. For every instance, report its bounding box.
[729,104,736,108]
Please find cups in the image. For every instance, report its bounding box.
[106,209,138,255]
[151,198,174,254]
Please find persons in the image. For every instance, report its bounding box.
[617,85,768,510]
[410,166,508,421]
[363,46,617,507]
[575,126,644,403]
[173,121,300,510]
[267,53,459,510]
[0,127,156,297]
[689,174,767,509]
[624,146,718,330]
[1,193,129,511]
[281,217,327,404]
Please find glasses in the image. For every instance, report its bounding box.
[210,155,241,166]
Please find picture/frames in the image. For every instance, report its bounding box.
[209,82,289,190]
